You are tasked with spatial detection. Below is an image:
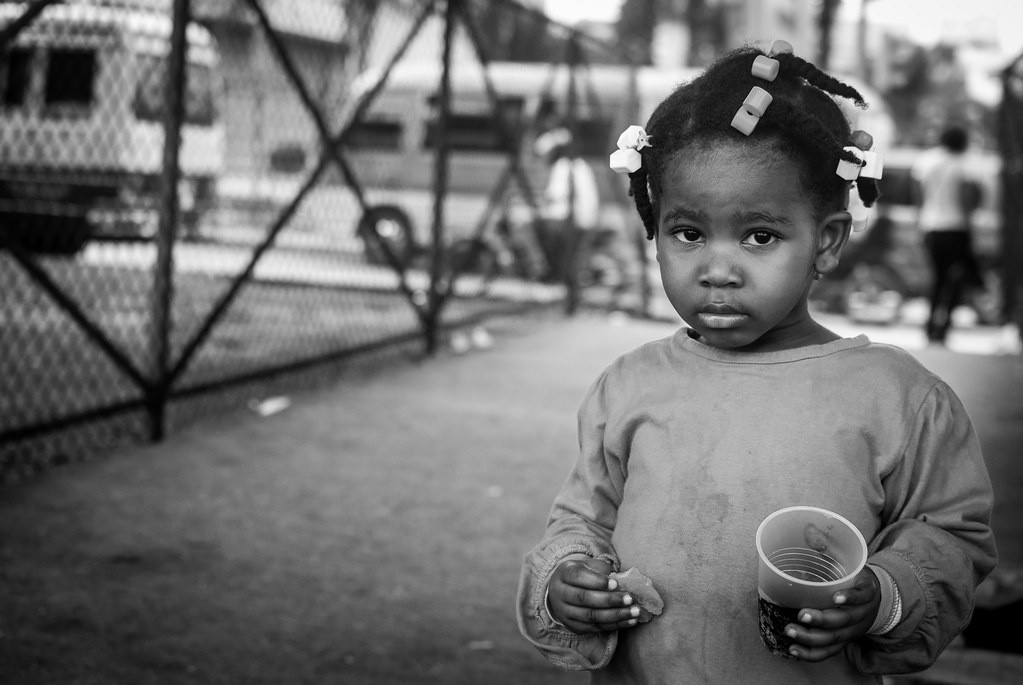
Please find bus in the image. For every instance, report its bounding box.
[327,55,897,274]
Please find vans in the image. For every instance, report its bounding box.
[824,144,1010,334]
[0,0,227,244]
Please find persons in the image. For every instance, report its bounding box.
[514,43,998,685]
[535,125,599,302]
[909,121,986,341]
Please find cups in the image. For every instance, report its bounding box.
[753,503,867,656]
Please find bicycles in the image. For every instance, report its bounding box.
[440,181,638,320]
